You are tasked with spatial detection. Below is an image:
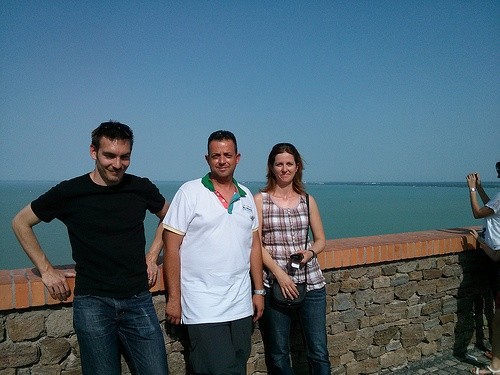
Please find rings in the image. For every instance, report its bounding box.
[149,278,153,281]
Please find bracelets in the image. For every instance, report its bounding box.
[308,250,314,259]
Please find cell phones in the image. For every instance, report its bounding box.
[290,255,302,260]
[467,174,477,180]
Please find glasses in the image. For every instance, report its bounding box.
[288,254,303,277]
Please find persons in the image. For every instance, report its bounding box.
[465,162,500,375]
[254,143,331,375]
[11,121,170,375]
[162,130,264,375]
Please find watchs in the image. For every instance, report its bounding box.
[469,187,477,192]
[253,289,266,296]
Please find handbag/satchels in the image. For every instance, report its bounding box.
[270,279,307,303]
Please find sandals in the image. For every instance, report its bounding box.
[470,365,500,375]
[484,351,493,360]
